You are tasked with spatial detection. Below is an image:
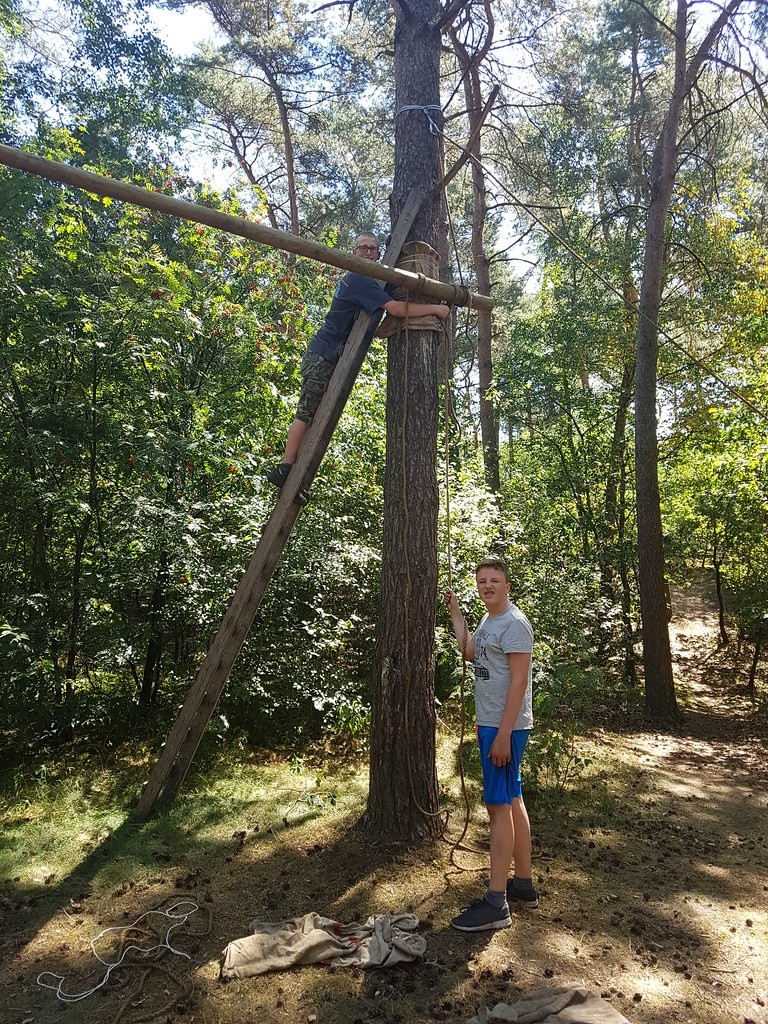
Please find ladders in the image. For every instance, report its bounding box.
[132,182,432,830]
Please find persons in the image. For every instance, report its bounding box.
[442,559,538,932]
[266,231,451,505]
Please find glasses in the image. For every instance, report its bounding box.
[355,245,379,254]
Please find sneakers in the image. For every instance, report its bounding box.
[450,897,512,932]
[484,878,539,908]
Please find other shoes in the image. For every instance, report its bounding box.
[268,463,311,505]
